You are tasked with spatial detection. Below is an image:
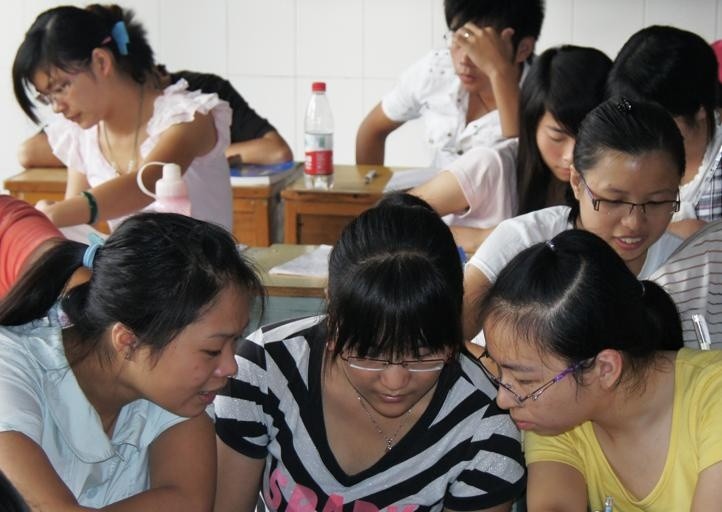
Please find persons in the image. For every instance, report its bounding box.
[215,191,528,512]
[18,3,294,169]
[1,194,92,300]
[357,1,545,173]
[401,25,721,351]
[13,6,233,238]
[473,228,721,511]
[2,211,268,512]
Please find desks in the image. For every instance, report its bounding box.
[280,164,424,245]
[3,160,304,249]
[239,243,340,298]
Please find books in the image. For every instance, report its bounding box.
[230,164,298,185]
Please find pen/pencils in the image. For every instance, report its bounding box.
[364,170,377,184]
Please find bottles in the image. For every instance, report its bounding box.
[137,161,192,218]
[303,82,336,189]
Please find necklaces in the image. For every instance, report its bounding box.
[104,83,144,176]
[355,386,412,455]
[105,407,120,436]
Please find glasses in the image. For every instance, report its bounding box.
[340,349,445,371]
[579,174,680,215]
[478,352,595,406]
[37,36,111,105]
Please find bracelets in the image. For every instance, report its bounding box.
[81,190,97,225]
